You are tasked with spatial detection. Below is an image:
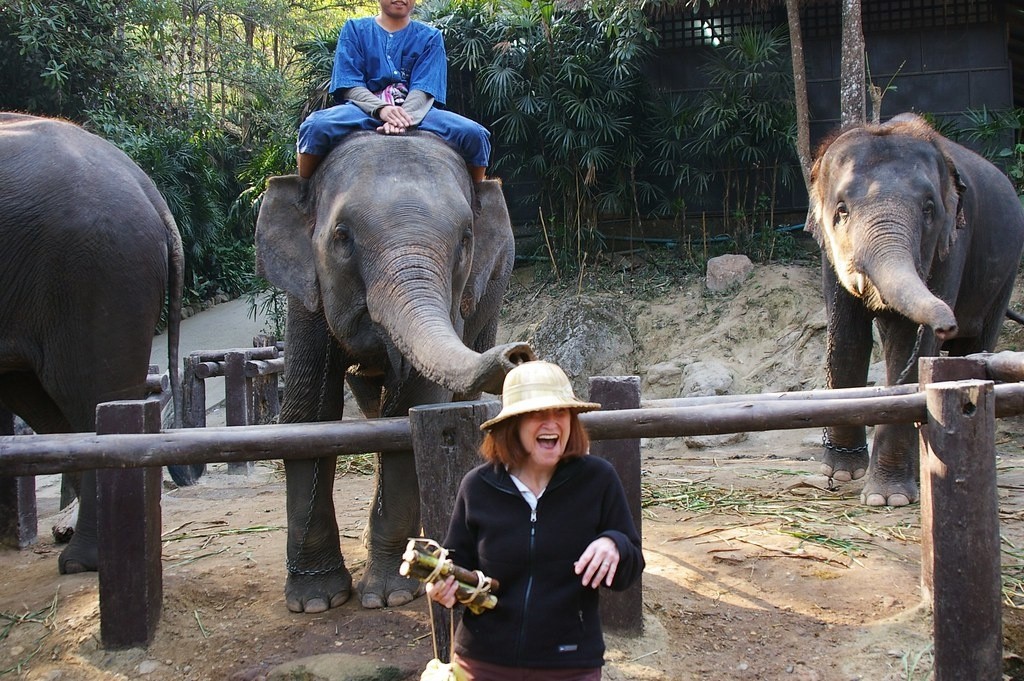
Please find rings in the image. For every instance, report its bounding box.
[602,562,610,566]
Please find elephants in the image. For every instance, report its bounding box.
[803,113,1024,503]
[0,112,184,574]
[255,130,536,613]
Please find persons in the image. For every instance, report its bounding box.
[296,0,492,183]
[425,360,647,681]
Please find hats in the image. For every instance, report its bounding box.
[480,361,601,432]
